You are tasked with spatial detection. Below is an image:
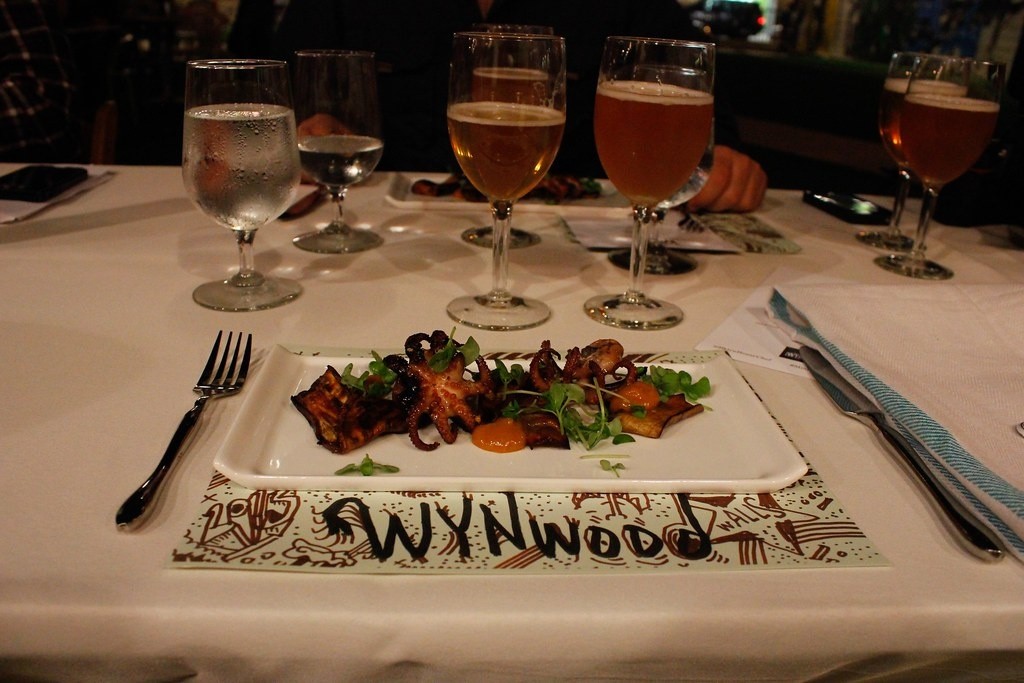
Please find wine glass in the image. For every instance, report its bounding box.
[607,65,715,275]
[854,54,970,255]
[583,36,715,330]
[292,49,385,254]
[873,55,1006,280]
[461,24,554,250]
[446,32,567,332]
[182,59,302,311]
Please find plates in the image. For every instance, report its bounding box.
[213,345,808,494]
[383,172,633,218]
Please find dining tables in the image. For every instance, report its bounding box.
[0,157,1024,683]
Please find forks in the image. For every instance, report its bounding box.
[114,330,252,533]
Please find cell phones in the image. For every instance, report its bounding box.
[803,187,893,226]
[0,165,88,202]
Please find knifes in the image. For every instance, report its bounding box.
[799,345,1003,562]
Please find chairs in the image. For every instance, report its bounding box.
[90,99,119,164]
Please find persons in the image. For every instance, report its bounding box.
[271,1,770,216]
[0,0,76,161]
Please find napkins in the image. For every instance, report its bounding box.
[763,273,1024,563]
[0,166,120,231]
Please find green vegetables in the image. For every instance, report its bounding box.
[457,168,603,205]
[334,325,713,478]
